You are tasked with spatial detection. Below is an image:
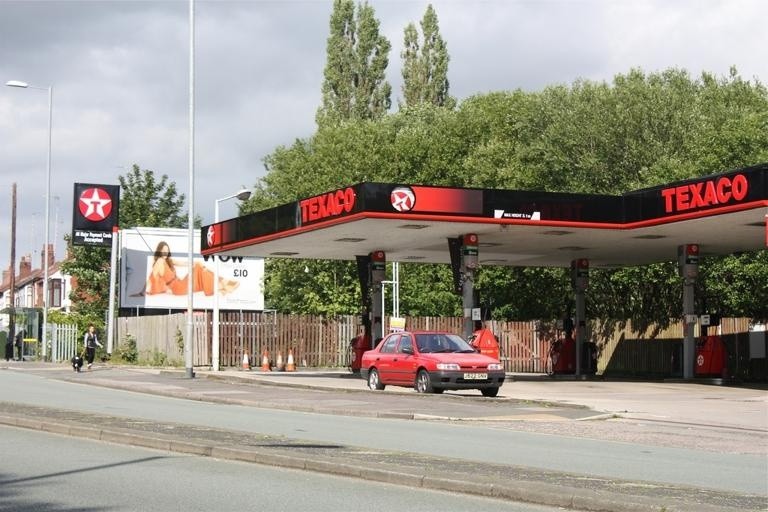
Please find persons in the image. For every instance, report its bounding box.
[128,241,240,297]
[14,324,27,361]
[83,324,98,369]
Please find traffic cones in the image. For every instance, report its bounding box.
[262,350,296,372]
[243,350,250,371]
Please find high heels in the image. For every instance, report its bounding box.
[218,279,240,295]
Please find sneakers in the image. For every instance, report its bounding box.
[87,363,92,368]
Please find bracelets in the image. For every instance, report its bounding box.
[140,293,144,296]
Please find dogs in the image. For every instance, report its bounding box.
[72,355,83,372]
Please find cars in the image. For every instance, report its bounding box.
[359,331,505,396]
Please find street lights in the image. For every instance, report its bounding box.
[212,189,251,371]
[6,80,53,362]
